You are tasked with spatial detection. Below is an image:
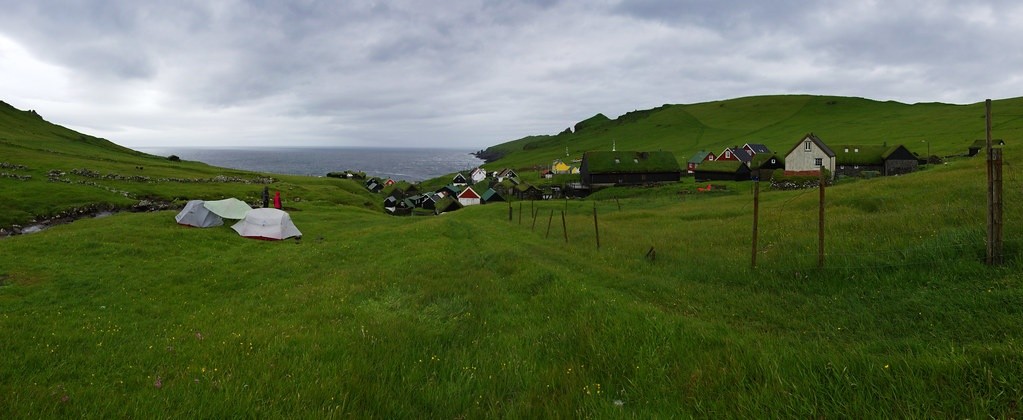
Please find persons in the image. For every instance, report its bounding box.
[260,186,269,208]
[274,192,282,209]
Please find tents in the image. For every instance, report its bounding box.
[230,207,302,240]
[175,200,224,227]
[204,198,252,219]
